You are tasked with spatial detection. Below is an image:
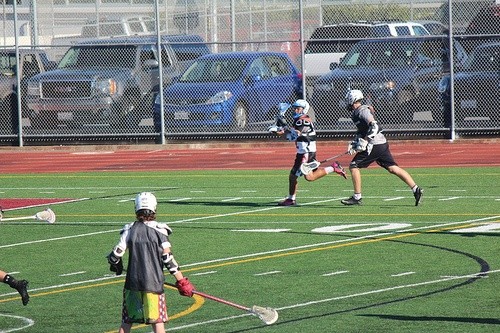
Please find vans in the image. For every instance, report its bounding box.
[81,14,158,37]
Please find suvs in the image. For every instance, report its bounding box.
[431,43,500,128]
[303,21,430,81]
[313,30,469,124]
[434,0,500,42]
[0,49,58,134]
[26,34,212,133]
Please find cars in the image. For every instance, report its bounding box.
[154,49,301,134]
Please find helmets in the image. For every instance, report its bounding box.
[134,192,158,213]
[293,99,310,119]
[343,90,364,111]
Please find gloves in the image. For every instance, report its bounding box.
[175,278,194,297]
[107,252,125,276]
[348,137,368,155]
[267,116,288,133]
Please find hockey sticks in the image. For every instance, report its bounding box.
[1,208,56,224]
[278,102,291,133]
[121,268,279,325]
[301,148,357,176]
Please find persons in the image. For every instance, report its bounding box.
[0,270,30,306]
[108,192,195,333]
[276,99,347,206]
[341,89,424,207]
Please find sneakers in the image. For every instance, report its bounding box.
[341,197,364,205]
[19,279,30,306]
[414,187,424,206]
[331,161,347,178]
[278,198,296,205]
